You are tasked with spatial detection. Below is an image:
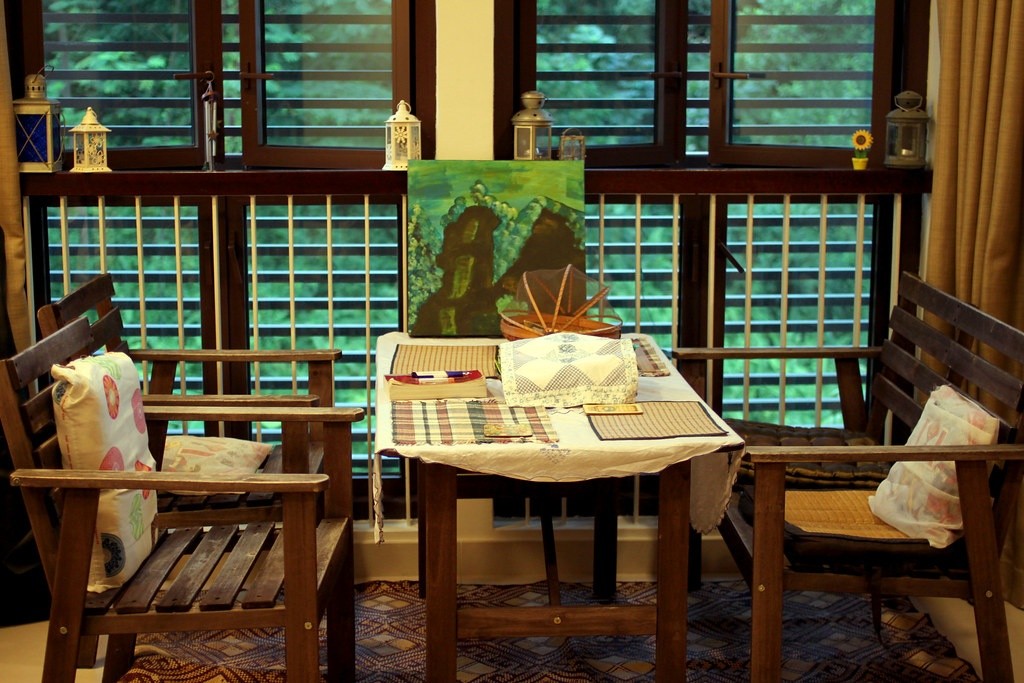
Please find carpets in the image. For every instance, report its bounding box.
[121,576,983,683]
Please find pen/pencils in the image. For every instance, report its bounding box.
[411,371,472,376]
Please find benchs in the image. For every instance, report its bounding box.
[689,271,1024,683]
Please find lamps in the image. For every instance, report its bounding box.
[512,91,554,162]
[12,65,67,173]
[382,100,422,171]
[883,90,930,169]
[558,127,585,164]
[67,106,112,173]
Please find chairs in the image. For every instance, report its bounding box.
[37,276,343,672]
[0,316,365,683]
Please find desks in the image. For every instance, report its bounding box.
[374,329,749,683]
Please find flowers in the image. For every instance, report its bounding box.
[852,130,873,158]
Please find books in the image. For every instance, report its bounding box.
[384,369,488,400]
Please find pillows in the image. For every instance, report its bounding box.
[868,386,1000,552]
[49,351,161,590]
[158,435,275,494]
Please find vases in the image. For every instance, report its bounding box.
[851,157,868,169]
